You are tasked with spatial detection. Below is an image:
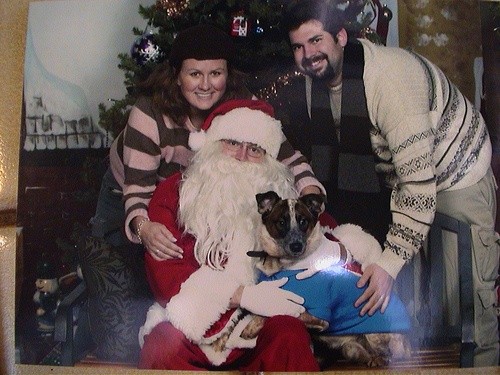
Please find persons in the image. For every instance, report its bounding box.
[138,98,384,371]
[80,24,328,363]
[279,3,499,367]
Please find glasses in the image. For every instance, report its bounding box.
[219,138,264,158]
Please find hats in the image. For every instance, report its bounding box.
[169,28,231,60]
[189,99,284,159]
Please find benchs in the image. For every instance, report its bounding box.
[54,189,478,368]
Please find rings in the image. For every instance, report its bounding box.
[155,249,160,255]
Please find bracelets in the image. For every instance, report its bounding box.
[137,218,149,246]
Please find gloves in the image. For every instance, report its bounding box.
[239,276,306,318]
[286,233,341,279]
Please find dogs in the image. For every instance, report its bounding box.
[255,190,412,369]
